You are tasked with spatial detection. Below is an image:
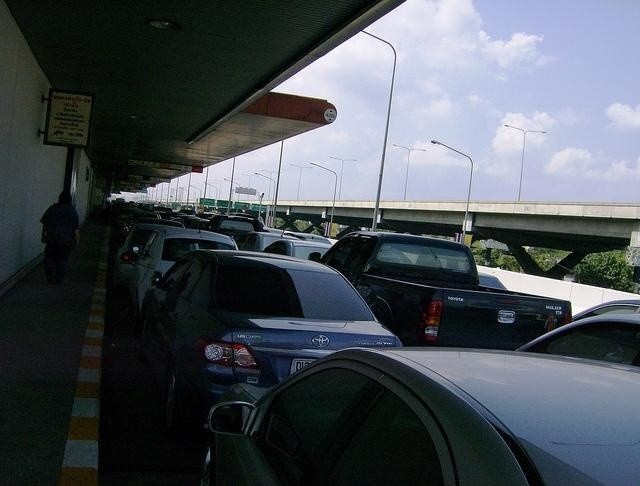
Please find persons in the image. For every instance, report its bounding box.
[40,190,82,286]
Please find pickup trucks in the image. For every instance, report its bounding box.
[321,231,571,346]
